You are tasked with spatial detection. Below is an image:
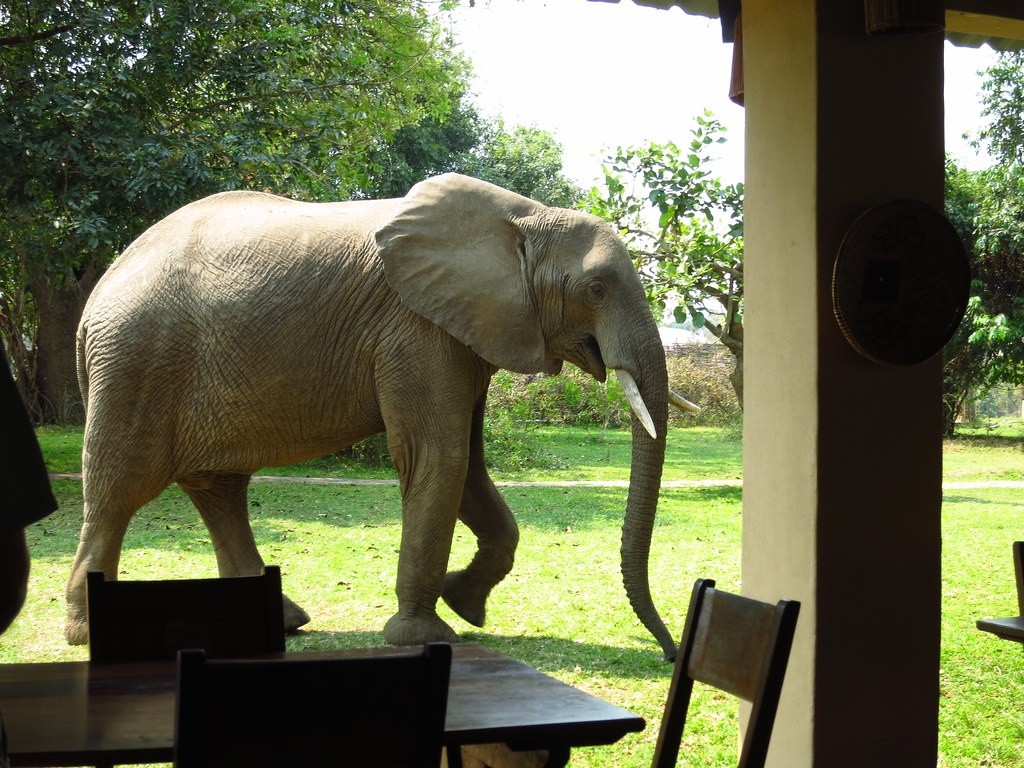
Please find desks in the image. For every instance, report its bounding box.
[1,640,645,768]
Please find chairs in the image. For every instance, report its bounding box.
[651,578,800,768]
[173,642,452,768]
[86,566,287,660]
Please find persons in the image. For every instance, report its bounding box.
[0,337,59,641]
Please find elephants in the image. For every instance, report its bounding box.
[67,173,701,662]
